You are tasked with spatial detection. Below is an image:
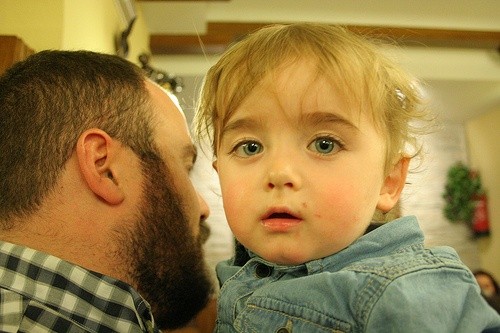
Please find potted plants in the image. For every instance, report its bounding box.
[442,162,489,236]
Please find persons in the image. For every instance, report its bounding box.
[472,269,499,309]
[204,21,500,333]
[0,49,217,332]
